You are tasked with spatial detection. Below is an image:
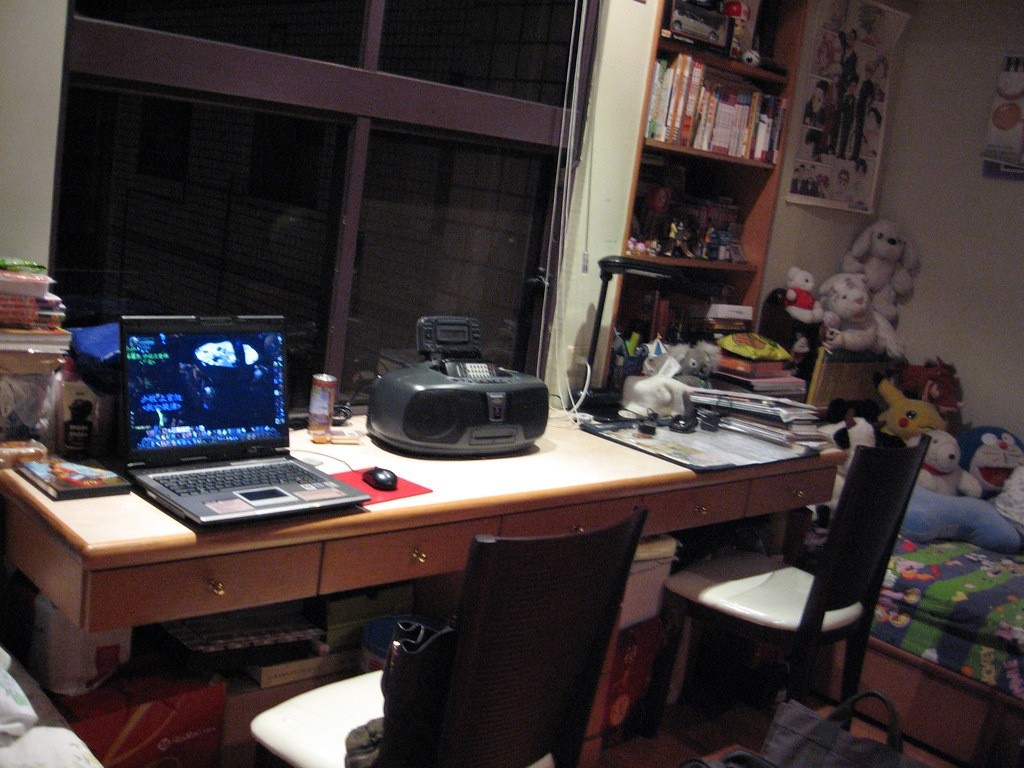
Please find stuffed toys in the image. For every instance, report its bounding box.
[806,354,1024,553]
[769,219,918,388]
[622,341,721,420]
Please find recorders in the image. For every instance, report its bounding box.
[366,315,549,457]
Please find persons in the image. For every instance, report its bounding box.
[664,218,715,259]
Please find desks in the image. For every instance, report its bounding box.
[0,415,848,632]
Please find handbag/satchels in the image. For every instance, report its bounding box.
[753,690,927,767]
[344,620,457,768]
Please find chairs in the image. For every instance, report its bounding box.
[667,435,932,734]
[249,505,650,768]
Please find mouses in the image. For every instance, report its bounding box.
[362,465,398,492]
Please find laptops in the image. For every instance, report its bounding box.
[120,314,371,529]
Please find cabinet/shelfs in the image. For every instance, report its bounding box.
[616,1,802,344]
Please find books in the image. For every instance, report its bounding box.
[626,292,755,341]
[644,53,788,165]
[18,456,131,500]
[687,359,828,445]
[0,268,73,355]
[166,621,365,691]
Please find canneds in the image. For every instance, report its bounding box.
[308,373,337,435]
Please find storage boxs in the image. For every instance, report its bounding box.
[46,665,225,768]
[623,535,676,629]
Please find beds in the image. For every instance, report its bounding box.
[801,513,1024,768]
